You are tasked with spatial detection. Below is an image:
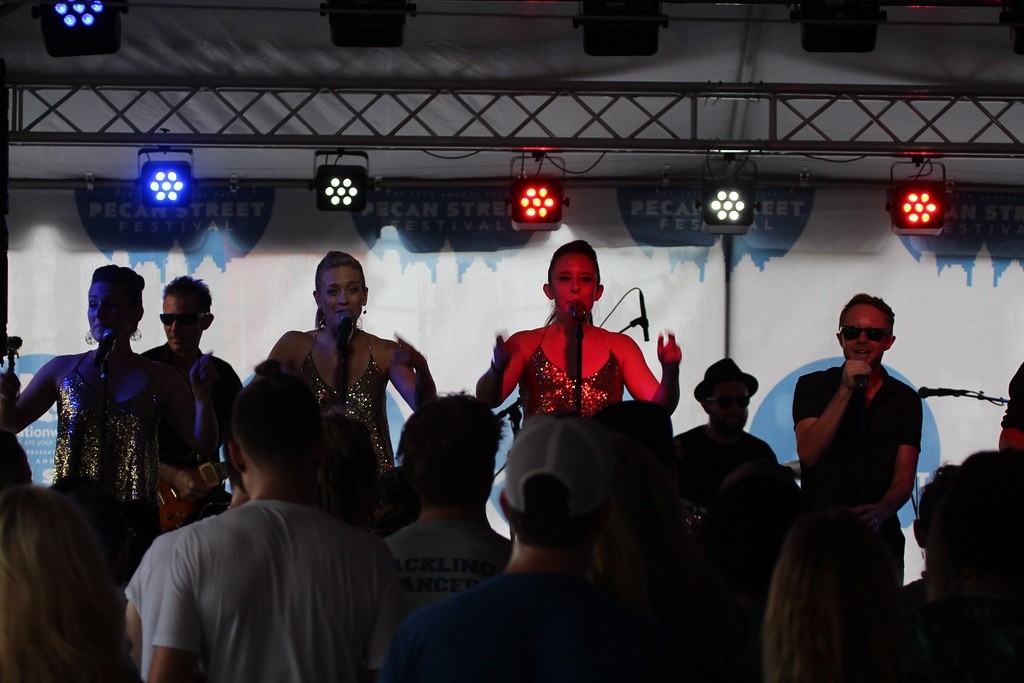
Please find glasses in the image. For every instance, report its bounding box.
[839,325,889,342]
[705,396,749,409]
[160,312,210,326]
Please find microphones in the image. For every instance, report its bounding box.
[855,376,868,391]
[639,289,649,341]
[569,299,586,322]
[919,386,969,400]
[93,328,118,365]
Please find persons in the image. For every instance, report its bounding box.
[138,275,244,532]
[316,411,380,531]
[762,506,937,683]
[999,362,1024,450]
[369,466,422,539]
[248,251,436,532]
[476,239,681,428]
[673,357,779,542]
[898,451,1024,683]
[0,265,219,581]
[380,399,759,683]
[124,374,510,683]
[793,293,922,584]
[0,429,145,683]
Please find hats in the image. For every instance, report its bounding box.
[506,411,612,517]
[694,357,759,403]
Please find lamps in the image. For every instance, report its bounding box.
[998,0,1024,54]
[789,1,888,54]
[29,0,130,59]
[307,147,375,213]
[504,152,569,232]
[696,152,761,235]
[886,155,953,238]
[321,0,418,49]
[573,0,670,56]
[136,145,199,208]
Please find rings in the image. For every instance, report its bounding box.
[875,518,878,526]
